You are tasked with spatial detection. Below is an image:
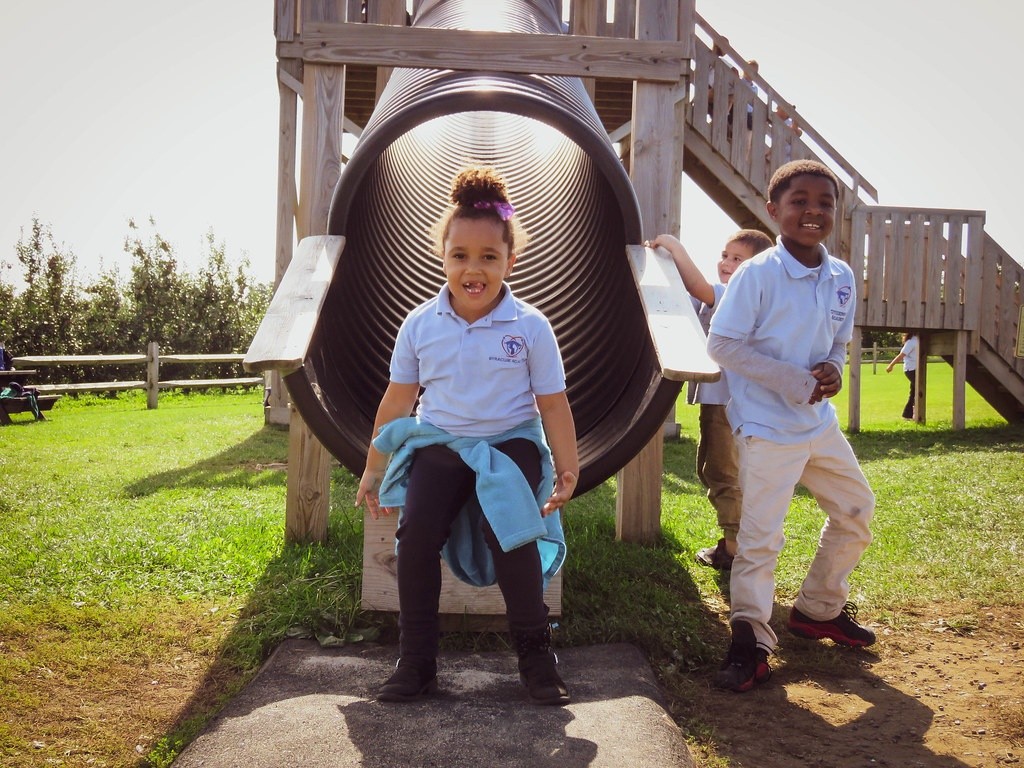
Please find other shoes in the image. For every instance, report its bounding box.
[695,539,733,571]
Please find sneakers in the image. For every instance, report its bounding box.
[786,605,876,647]
[376,652,439,702]
[718,620,773,692]
[518,653,571,705]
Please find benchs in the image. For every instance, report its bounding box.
[0,396,59,413]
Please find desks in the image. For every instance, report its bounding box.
[0,370,45,426]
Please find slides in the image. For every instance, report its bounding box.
[242,0,721,506]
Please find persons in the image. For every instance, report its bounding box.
[706,158,877,692]
[650,229,775,573]
[886,330,919,421]
[353,164,581,706]
